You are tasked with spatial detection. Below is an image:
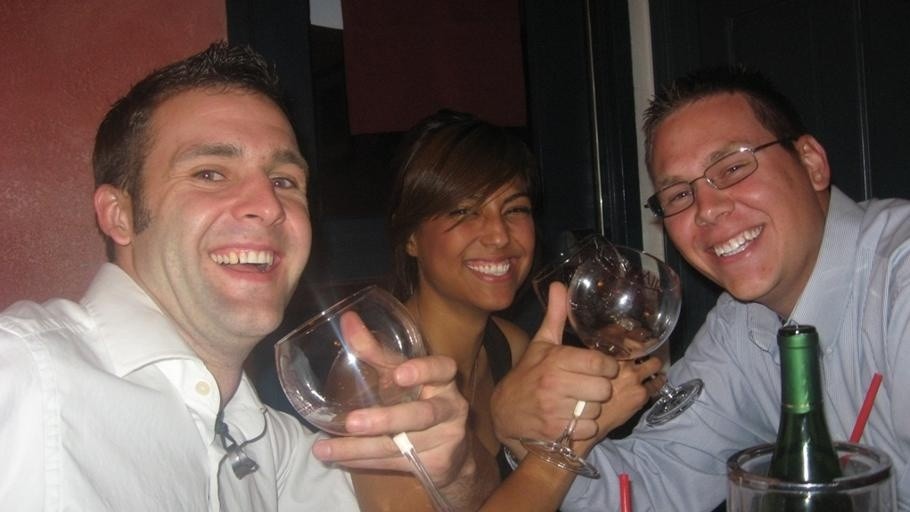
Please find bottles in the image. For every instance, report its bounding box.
[775,326,843,482]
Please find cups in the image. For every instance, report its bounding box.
[725,438,895,510]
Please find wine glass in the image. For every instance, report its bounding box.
[272,286,453,507]
[531,246,703,428]
[519,246,683,479]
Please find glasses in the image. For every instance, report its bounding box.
[643,136,793,218]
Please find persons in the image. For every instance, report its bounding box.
[0,38,503,511]
[319,107,668,512]
[487,67,910,511]
